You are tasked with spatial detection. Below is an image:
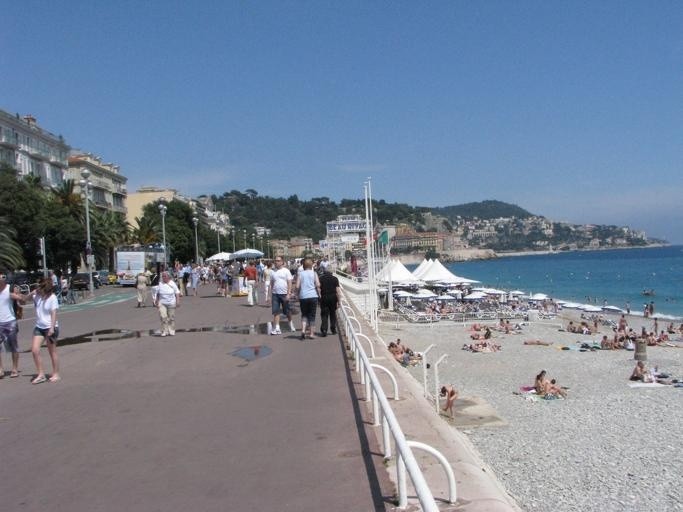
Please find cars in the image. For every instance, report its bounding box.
[72,273,101,290]
[100,271,117,287]
[92,271,102,284]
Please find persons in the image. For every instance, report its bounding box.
[50,269,76,305]
[136,257,341,340]
[1,269,24,378]
[387,298,683,418]
[25,279,61,384]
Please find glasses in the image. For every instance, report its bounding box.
[0,275,6,280]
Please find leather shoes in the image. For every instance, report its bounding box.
[300,325,337,340]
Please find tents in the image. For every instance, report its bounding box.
[377,257,457,284]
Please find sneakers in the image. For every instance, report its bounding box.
[271,329,281,335]
[160,331,169,337]
[288,327,295,332]
[169,328,175,336]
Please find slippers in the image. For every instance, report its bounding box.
[0,370,61,384]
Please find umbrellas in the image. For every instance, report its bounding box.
[231,247,263,260]
[206,252,231,261]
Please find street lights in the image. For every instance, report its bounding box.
[78,169,96,295]
[231,225,236,253]
[191,210,202,268]
[242,230,271,260]
[363,175,378,333]
[156,197,169,277]
[216,221,222,255]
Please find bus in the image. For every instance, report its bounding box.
[116,244,164,286]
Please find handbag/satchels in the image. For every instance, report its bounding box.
[10,283,23,320]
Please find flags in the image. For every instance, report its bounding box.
[371,231,378,241]
[380,232,389,244]
